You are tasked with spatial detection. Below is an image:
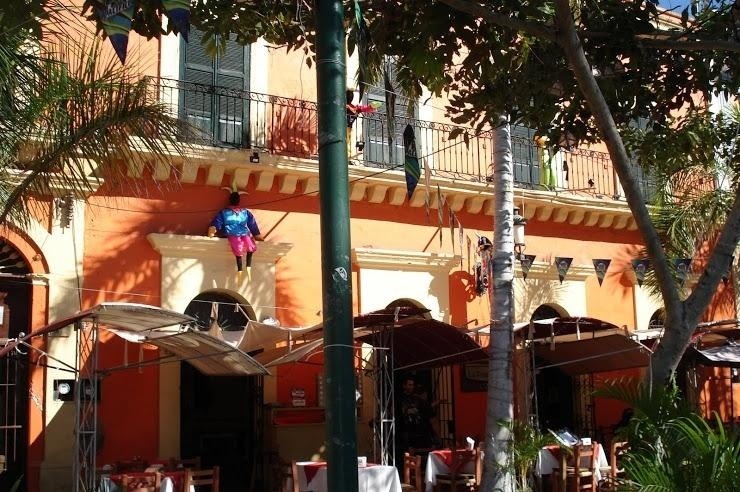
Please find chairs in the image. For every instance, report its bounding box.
[117,456,219,492]
[436,447,482,491]
[400,452,424,492]
[553,441,596,491]
[269,450,301,491]
[599,441,632,491]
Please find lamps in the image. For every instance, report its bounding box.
[476,236,493,291]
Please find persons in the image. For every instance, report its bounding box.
[399,376,447,481]
[347,90,359,165]
[208,192,265,285]
[616,408,634,428]
